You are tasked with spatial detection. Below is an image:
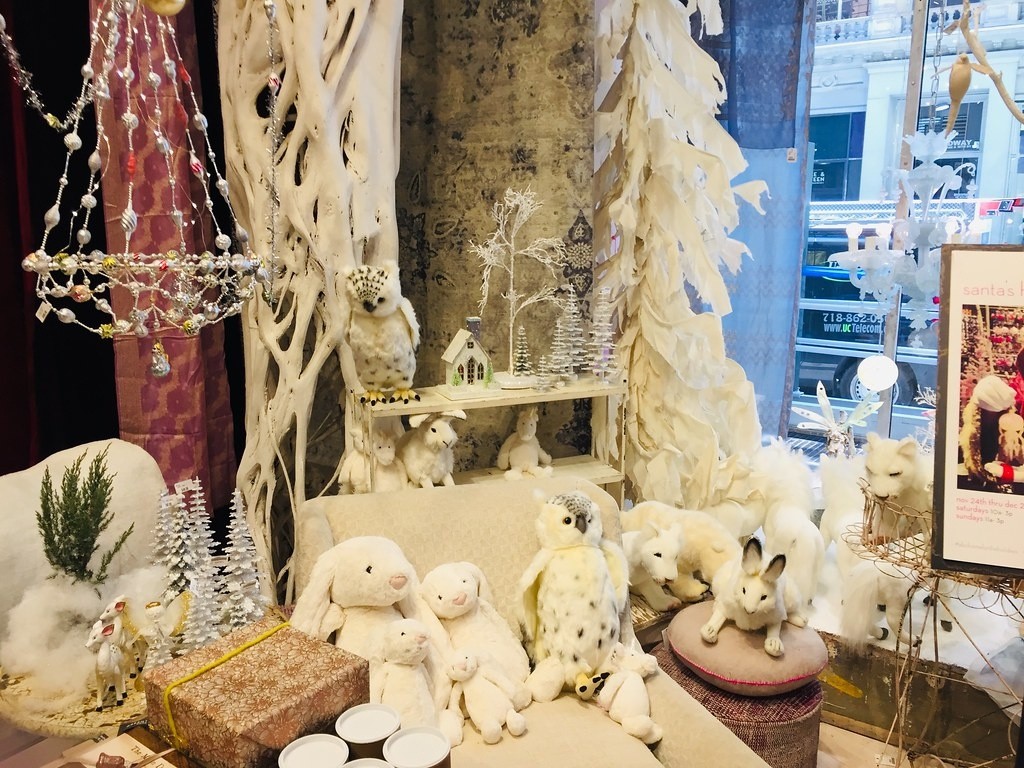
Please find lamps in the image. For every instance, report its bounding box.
[827,0,987,392]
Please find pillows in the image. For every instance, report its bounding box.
[662,600,828,696]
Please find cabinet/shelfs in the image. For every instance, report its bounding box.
[350,375,627,510]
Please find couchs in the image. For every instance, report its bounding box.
[294,475,771,768]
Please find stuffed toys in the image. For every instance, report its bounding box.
[283,263,953,745]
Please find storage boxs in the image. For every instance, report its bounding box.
[143,615,368,768]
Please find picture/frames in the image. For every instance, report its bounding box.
[930,243,1024,578]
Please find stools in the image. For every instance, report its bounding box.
[652,644,823,768]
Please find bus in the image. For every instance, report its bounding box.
[797,196,1024,406]
